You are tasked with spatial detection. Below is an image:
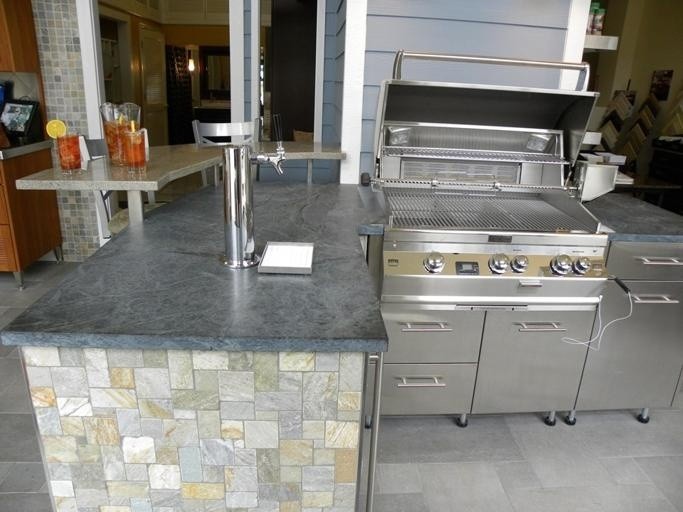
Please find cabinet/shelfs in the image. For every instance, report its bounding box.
[1,0,64,291]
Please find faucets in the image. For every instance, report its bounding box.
[222,141,285,269]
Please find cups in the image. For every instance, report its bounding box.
[55,128,82,175]
[100,101,145,174]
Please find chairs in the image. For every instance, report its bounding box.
[191,118,259,186]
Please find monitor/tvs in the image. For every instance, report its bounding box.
[0,80,14,112]
[0,99,44,146]
